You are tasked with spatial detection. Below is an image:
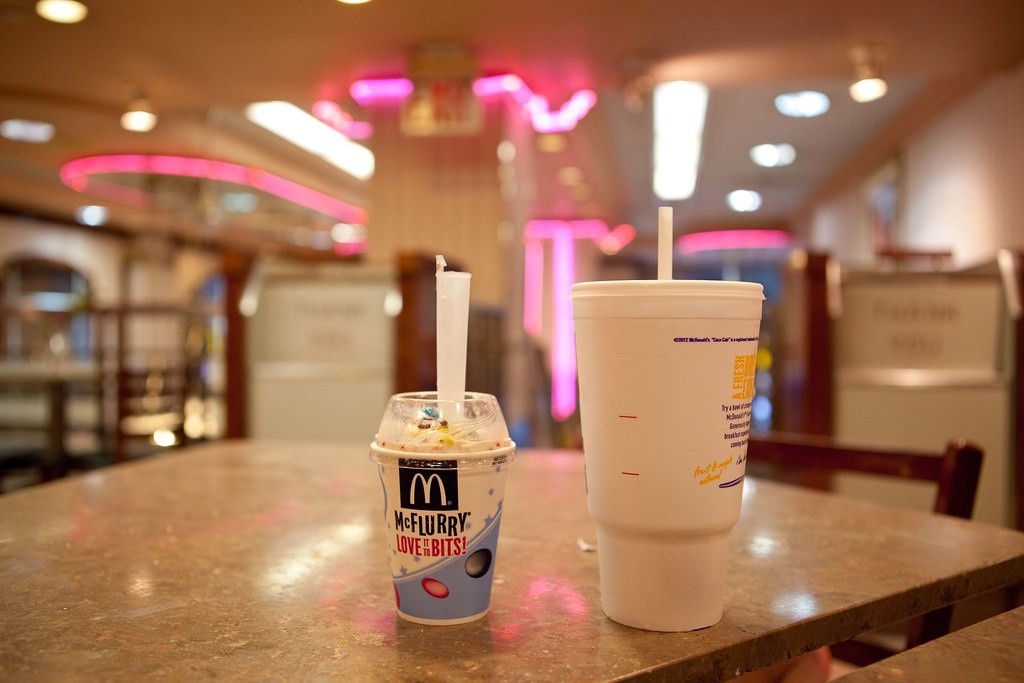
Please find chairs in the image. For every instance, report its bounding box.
[742,432,986,662]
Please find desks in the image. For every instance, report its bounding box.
[0,442,1024,683]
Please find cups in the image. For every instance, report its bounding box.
[571,280,765,632]
[372,392,517,625]
